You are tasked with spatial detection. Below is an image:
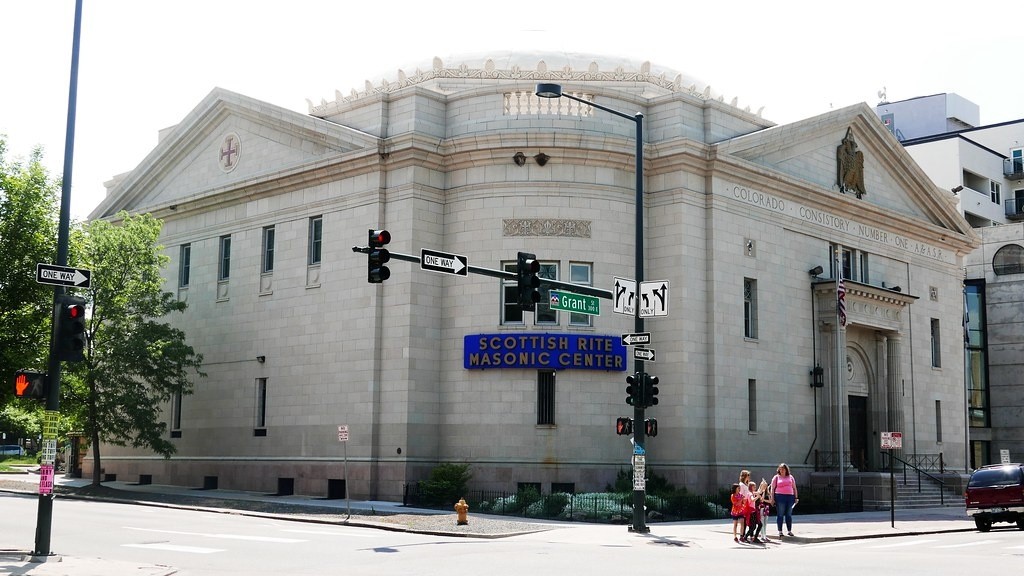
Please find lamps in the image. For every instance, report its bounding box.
[809,264,823,279]
[809,364,824,388]
[888,285,901,292]
[257,356,265,364]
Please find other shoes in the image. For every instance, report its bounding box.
[739,538,745,543]
[788,532,794,536]
[753,538,763,543]
[733,537,740,543]
[779,534,784,537]
[760,538,770,542]
[744,537,750,543]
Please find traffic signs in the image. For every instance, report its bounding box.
[420,248,468,277]
[36,263,91,288]
[621,332,651,346]
[634,348,656,362]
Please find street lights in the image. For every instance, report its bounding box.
[536,83,652,532]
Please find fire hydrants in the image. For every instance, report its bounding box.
[454,496,469,525]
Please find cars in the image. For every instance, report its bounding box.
[0,445,36,458]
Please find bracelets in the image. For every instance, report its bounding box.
[795,497,798,499]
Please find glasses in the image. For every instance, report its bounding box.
[747,474,749,476]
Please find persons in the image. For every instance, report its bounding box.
[742,482,764,544]
[739,470,754,539]
[769,463,798,537]
[757,480,772,542]
[731,484,746,541]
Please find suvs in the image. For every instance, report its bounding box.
[965,462,1024,532]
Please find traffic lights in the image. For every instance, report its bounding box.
[645,416,658,438]
[53,293,85,360]
[13,370,48,399]
[518,252,541,311]
[626,371,640,406]
[368,229,390,283]
[616,417,631,435]
[643,373,659,406]
